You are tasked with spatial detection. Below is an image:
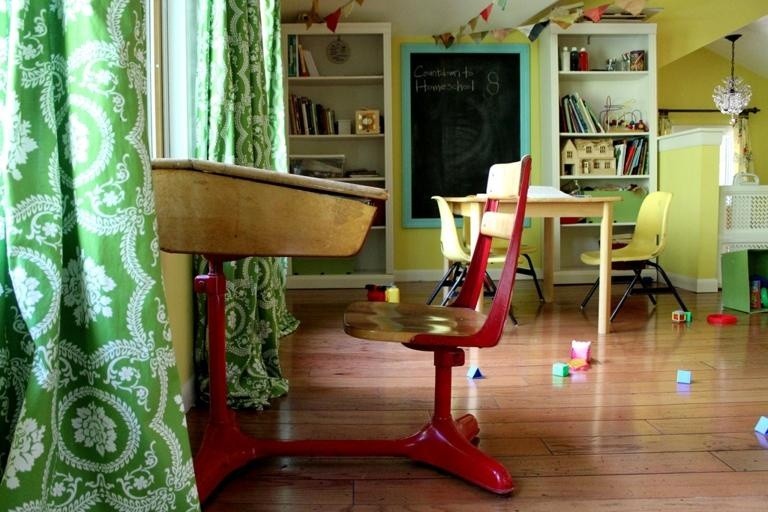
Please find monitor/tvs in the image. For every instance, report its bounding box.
[289,94,337,135]
[345,168,380,178]
[288,36,322,77]
[560,92,604,132]
[615,137,649,175]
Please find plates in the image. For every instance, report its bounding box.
[281,21,393,289]
[546,21,659,286]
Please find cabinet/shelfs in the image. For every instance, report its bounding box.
[599,105,626,132]
[658,109,671,134]
[751,279,761,309]
[560,46,589,71]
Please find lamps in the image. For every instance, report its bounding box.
[426,195,525,326]
[463,219,546,304]
[340,154,533,495]
[579,192,689,322]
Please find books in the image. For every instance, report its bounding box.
[722,249,768,315]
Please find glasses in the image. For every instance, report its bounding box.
[712,33,752,124]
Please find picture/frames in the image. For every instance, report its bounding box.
[439,195,624,335]
[153,158,387,507]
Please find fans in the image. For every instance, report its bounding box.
[400,43,531,229]
